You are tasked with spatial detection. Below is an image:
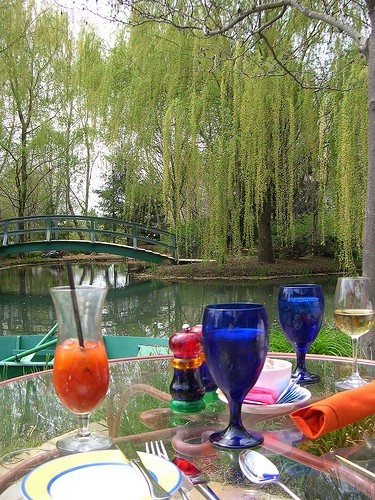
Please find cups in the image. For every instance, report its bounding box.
[252,359,294,399]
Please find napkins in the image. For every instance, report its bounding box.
[289,379,375,440]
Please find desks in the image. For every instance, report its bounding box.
[0,350,375,500]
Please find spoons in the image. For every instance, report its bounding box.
[238,449,303,500]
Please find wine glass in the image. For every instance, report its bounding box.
[277,284,325,384]
[334,277,375,390]
[203,302,270,448]
[48,285,116,453]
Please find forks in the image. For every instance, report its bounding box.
[145,440,194,500]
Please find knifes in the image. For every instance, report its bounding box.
[172,456,222,500]
[117,438,170,500]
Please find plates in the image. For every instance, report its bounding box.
[22,449,182,500]
[217,384,310,415]
